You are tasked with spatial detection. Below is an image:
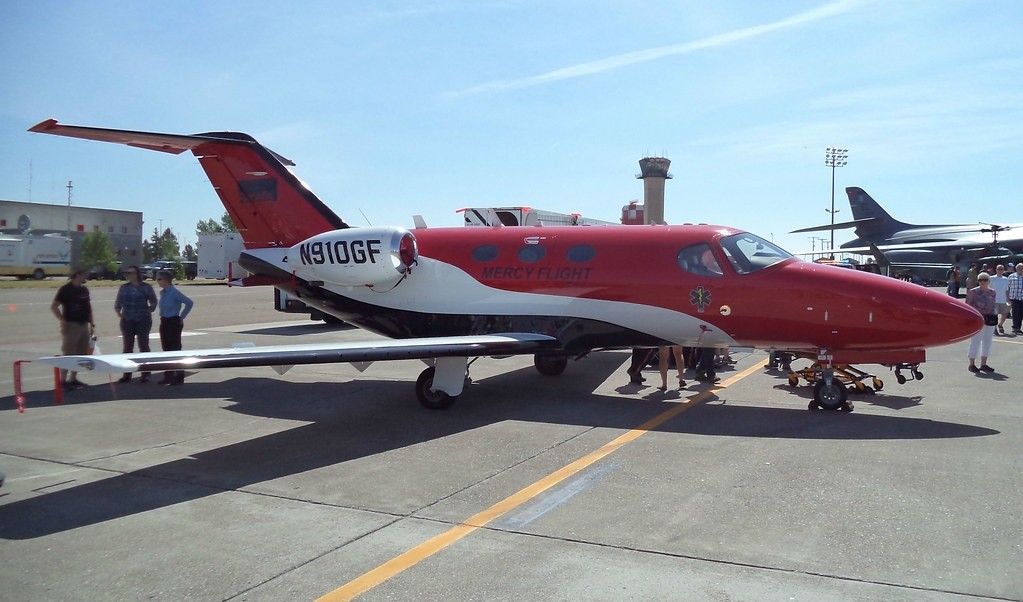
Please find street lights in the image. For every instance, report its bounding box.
[824,146,849,260]
[808,237,830,261]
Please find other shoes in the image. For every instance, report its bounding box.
[969,365,980,373]
[723,356,737,364]
[158,375,175,384]
[694,374,707,381]
[60,381,74,392]
[140,375,150,382]
[660,386,667,390]
[118,375,131,382]
[626,367,646,382]
[169,376,184,385]
[713,357,724,365]
[630,378,642,384]
[994,327,1023,336]
[68,379,88,389]
[706,375,721,382]
[679,381,687,387]
[979,364,994,372]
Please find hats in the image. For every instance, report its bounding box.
[67,264,84,278]
[1009,262,1015,267]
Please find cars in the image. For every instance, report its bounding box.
[87,261,124,280]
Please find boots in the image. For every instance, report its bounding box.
[764,356,779,368]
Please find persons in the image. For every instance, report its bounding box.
[966,272,998,373]
[155,270,194,386]
[626,346,737,390]
[945,262,1023,336]
[51,264,96,391]
[114,265,158,383]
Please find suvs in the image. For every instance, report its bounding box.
[179,261,198,281]
[139,260,178,280]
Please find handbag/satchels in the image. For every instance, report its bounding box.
[982,313,998,327]
[85,334,102,356]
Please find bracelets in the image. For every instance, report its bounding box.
[91,324,95,327]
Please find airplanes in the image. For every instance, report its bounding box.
[788,185,1023,295]
[10,117,985,412]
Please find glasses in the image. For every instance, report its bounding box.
[157,278,166,281]
[127,272,136,275]
[978,279,989,282]
